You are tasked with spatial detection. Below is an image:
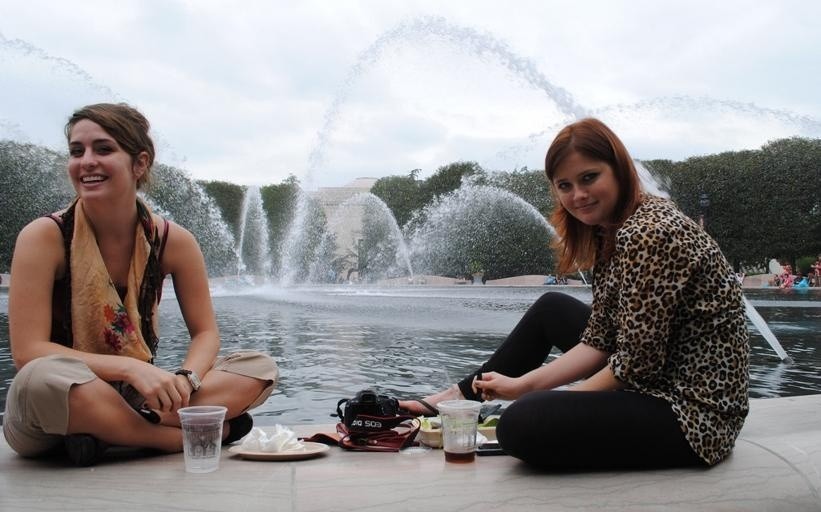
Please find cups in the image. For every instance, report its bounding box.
[438,399,483,463]
[178,405,229,474]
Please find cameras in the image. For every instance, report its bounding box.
[341,388,400,428]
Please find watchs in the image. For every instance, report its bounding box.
[174,369,202,395]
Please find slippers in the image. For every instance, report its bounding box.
[398,398,439,418]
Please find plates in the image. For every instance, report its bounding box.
[230,442,332,460]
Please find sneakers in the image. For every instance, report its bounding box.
[64,433,97,464]
[222,414,252,446]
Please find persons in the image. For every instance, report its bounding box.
[0,102,279,465]
[399,115,751,475]
[733,261,821,289]
